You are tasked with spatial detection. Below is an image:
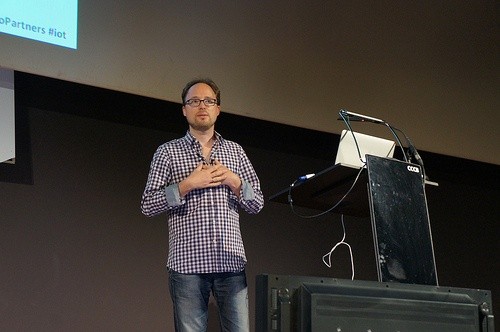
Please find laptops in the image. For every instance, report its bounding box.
[336,130,395,173]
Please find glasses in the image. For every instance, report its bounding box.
[183,98,218,106]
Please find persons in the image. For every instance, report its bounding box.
[140,80,264,332]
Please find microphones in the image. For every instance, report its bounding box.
[298,173,316,181]
[403,145,415,162]
